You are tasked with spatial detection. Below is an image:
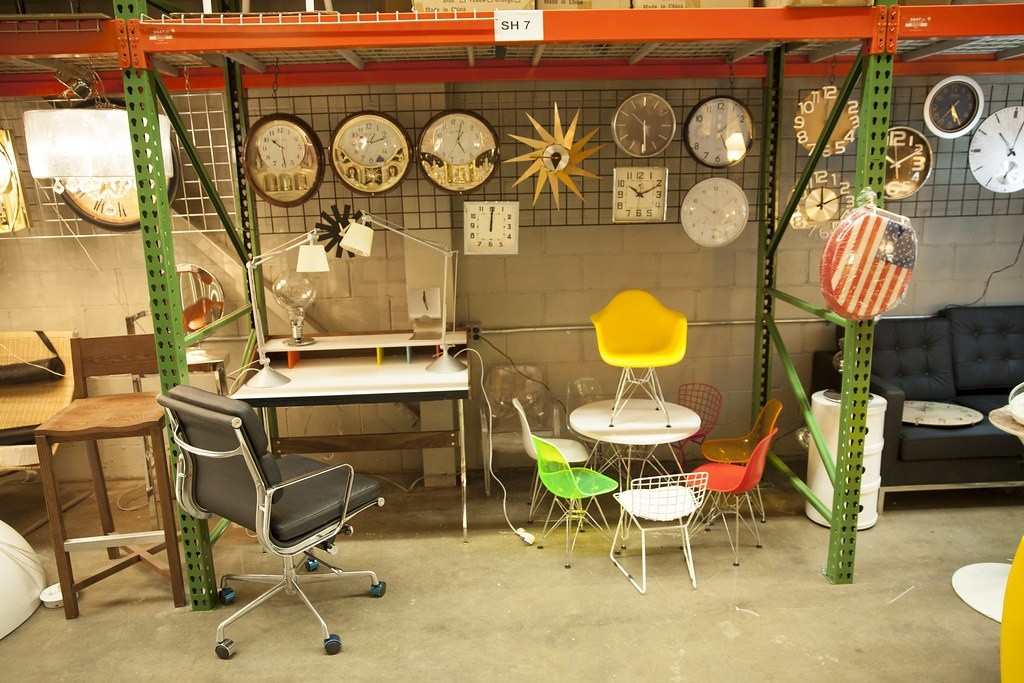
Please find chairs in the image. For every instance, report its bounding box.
[702,398,783,525]
[639,383,722,480]
[478,364,560,497]
[156,384,387,660]
[512,398,589,532]
[531,434,622,568]
[589,290,687,428]
[679,427,778,568]
[609,472,709,595]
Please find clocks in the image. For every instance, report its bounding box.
[885,126,933,200]
[503,102,607,211]
[684,95,755,168]
[415,110,501,195]
[612,167,668,223]
[968,106,1024,193]
[328,110,414,197]
[463,200,520,256]
[923,75,984,139]
[241,112,326,208]
[792,85,860,156]
[680,177,749,247]
[786,170,855,239]
[52,103,180,233]
[315,204,371,258]
[611,93,676,157]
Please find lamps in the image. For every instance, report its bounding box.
[340,210,468,374]
[245,228,330,388]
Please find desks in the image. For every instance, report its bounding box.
[569,399,701,550]
[223,330,477,547]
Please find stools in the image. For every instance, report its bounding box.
[34,333,186,621]
[125,264,230,530]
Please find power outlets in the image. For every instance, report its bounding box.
[471,322,482,342]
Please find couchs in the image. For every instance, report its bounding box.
[809,305,1024,515]
